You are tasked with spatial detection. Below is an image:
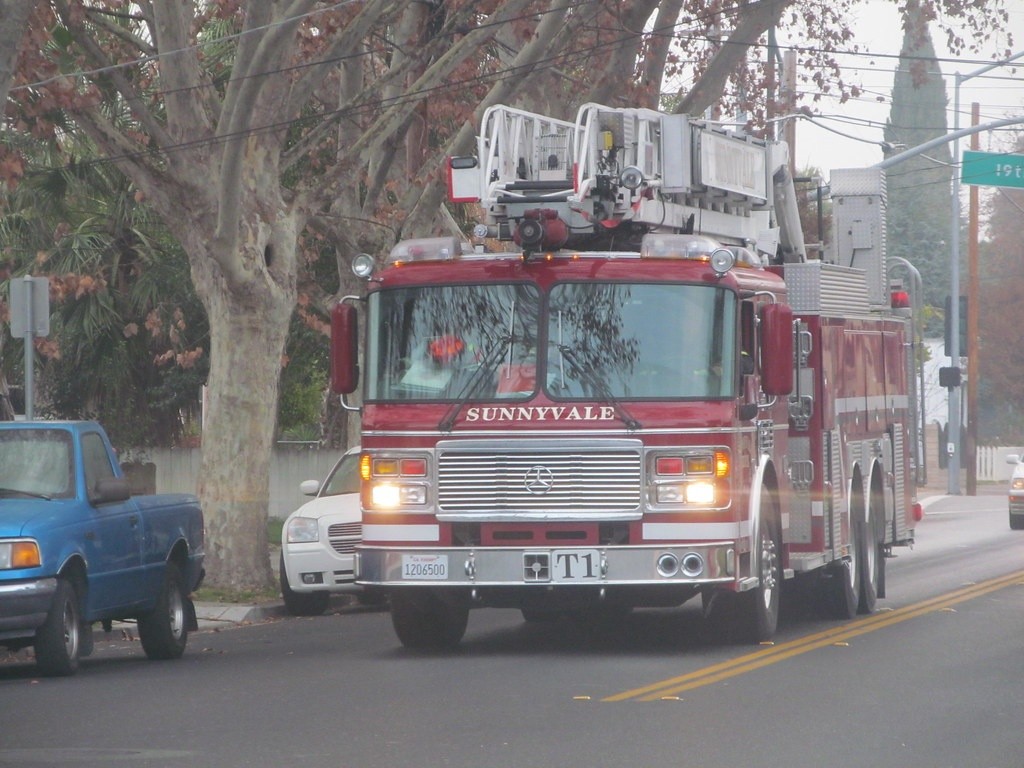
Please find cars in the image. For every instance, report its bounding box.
[278,446,362,615]
[1005,450,1024,530]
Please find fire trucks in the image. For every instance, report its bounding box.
[326,102,921,648]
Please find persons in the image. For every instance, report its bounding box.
[645,303,754,378]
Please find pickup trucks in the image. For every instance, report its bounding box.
[0,418,207,678]
